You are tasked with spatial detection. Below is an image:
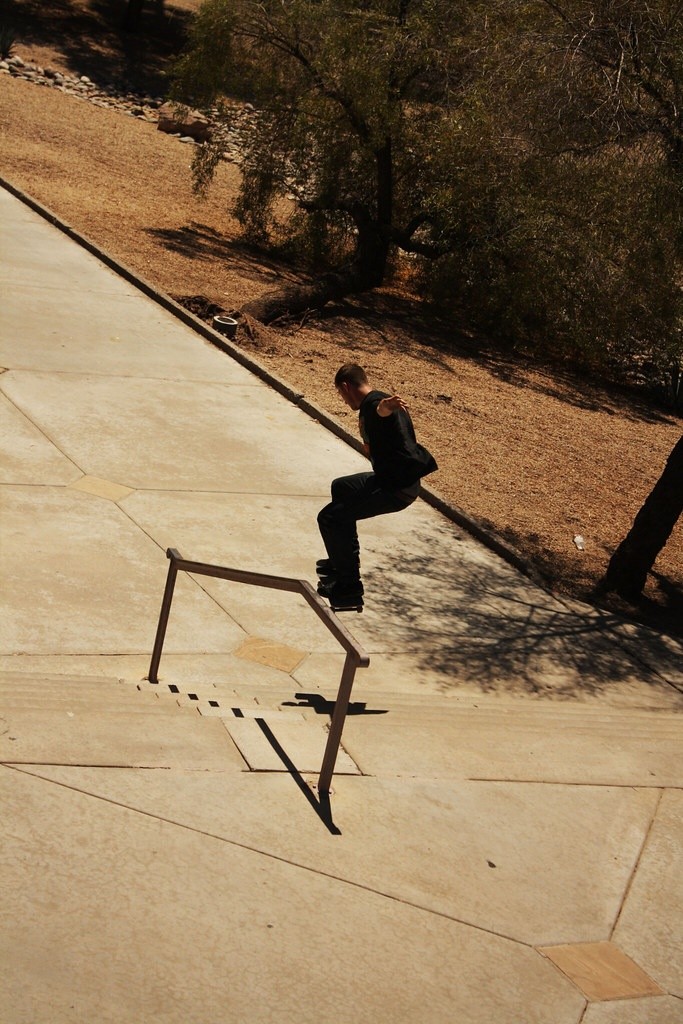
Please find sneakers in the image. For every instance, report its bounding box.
[317,555,360,576]
[318,581,365,598]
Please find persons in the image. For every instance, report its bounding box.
[317,364,419,598]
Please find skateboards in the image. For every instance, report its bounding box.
[316,559,368,613]
